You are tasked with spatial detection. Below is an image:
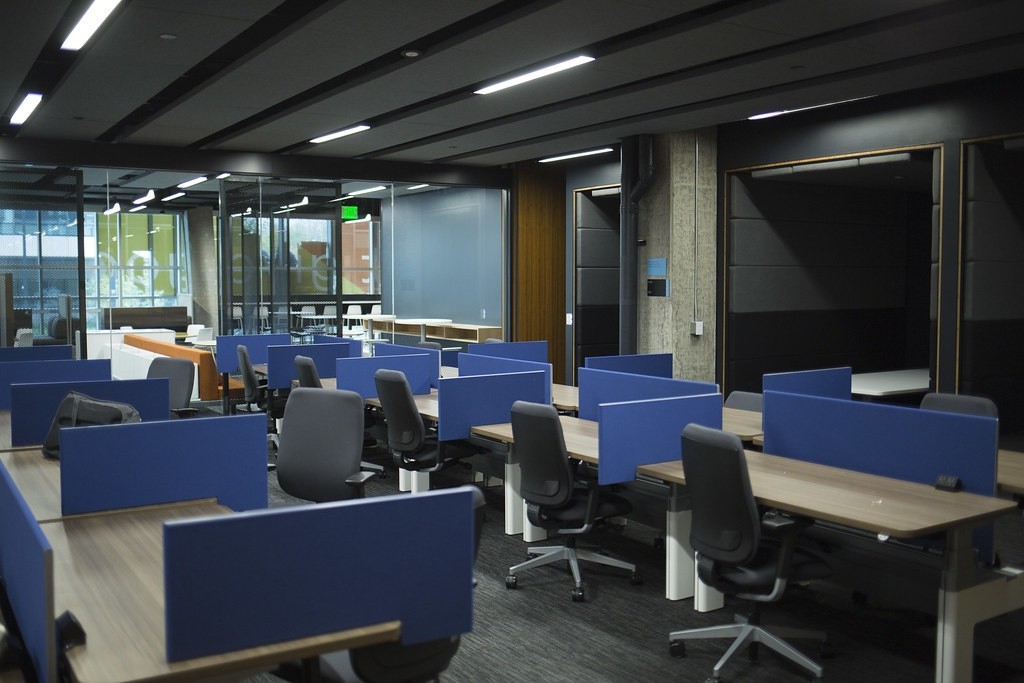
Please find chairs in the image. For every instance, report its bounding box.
[505,398,641,601]
[372,368,487,489]
[318,305,338,336]
[264,387,385,506]
[670,423,834,683]
[146,357,199,419]
[232,307,243,335]
[294,354,323,389]
[342,305,362,335]
[362,305,381,329]
[259,306,271,333]
[236,345,278,415]
[295,306,316,329]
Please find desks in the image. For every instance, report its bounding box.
[0,319,1024,683]
